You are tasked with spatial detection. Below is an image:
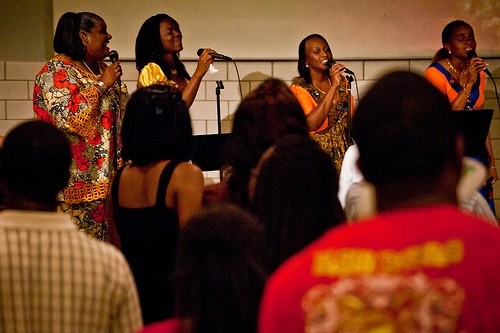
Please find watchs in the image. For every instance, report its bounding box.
[95,81,107,91]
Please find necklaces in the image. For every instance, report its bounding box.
[446,57,470,75]
[82,58,99,76]
[312,75,332,95]
[103,85,204,325]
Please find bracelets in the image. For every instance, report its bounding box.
[489,161,496,168]
[463,83,471,98]
[91,82,103,95]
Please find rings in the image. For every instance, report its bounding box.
[114,69,118,73]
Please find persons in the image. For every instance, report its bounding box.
[0,119,143,333]
[32,12,131,244]
[258,71,500,333]
[249,133,345,276]
[422,20,498,217]
[205,78,309,211]
[289,33,356,177]
[135,13,218,111]
[177,202,275,333]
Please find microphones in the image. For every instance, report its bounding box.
[326,59,354,75]
[467,50,492,76]
[109,50,122,87]
[197,49,232,61]
